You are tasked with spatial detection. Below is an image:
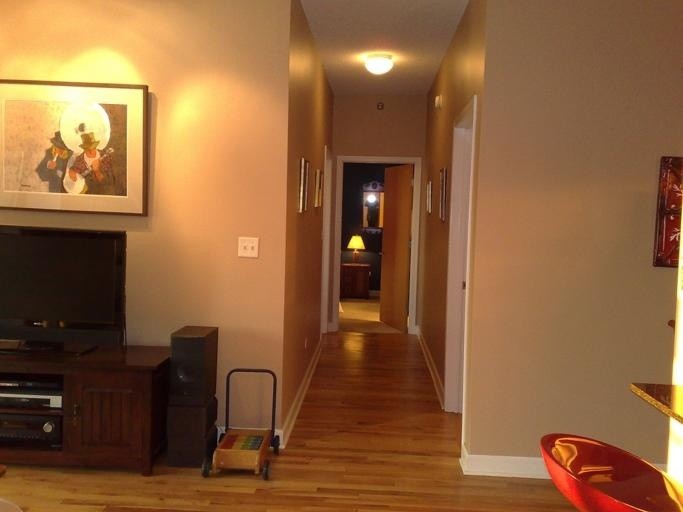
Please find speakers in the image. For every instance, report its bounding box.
[170,326,218,404]
[166,398,217,468]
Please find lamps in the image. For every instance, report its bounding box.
[347,235,365,263]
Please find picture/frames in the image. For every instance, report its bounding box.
[0,78,149,217]
[651,157,682,268]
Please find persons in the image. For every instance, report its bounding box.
[35,131,73,193]
[69,133,117,194]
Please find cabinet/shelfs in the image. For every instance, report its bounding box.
[340,262,373,299]
[0,340,172,476]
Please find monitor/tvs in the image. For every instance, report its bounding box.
[0,225,125,354]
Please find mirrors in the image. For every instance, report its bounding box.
[358,180,384,235]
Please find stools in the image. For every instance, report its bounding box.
[538,432,683,512]
